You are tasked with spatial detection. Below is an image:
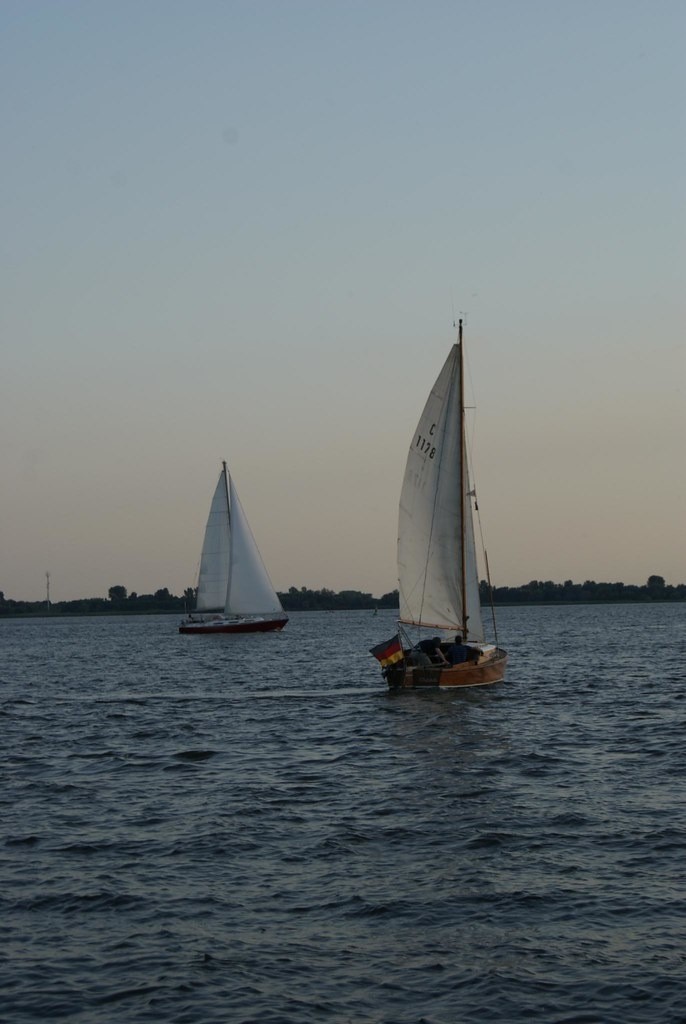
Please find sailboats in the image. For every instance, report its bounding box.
[175,460,291,635]
[368,317,514,688]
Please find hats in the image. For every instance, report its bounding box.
[455,635,462,640]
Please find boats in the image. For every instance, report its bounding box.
[371,600,382,620]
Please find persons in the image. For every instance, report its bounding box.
[449,636,468,664]
[429,637,449,664]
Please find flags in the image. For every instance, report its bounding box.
[370,635,404,667]
